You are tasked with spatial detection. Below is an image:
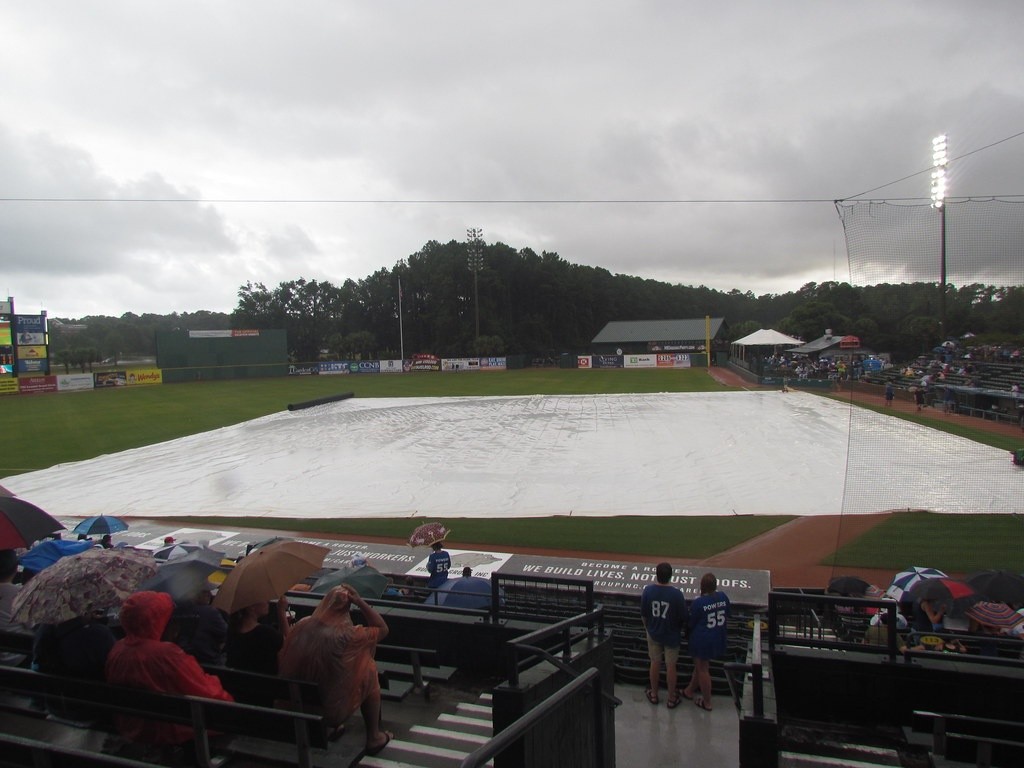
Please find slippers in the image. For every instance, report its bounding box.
[329,724,345,740]
[366,730,395,756]
[694,697,712,712]
[676,688,693,700]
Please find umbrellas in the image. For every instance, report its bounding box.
[423,575,505,608]
[821,564,1024,635]
[154,540,210,561]
[248,537,294,556]
[158,547,225,597]
[0,497,158,629]
[213,541,330,622]
[312,565,390,602]
[932,347,945,353]
[941,341,955,347]
[406,521,450,549]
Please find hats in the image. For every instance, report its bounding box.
[164,537,176,543]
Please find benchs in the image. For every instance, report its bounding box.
[912,707,1024,768]
[0,589,456,768]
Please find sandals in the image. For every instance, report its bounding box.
[667,696,681,709]
[646,689,658,704]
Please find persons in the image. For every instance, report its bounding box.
[885,381,894,406]
[863,611,925,654]
[452,363,459,373]
[21,330,32,343]
[675,572,733,711]
[749,350,887,383]
[899,342,1024,422]
[425,544,451,589]
[639,563,686,709]
[914,598,943,632]
[0,530,415,755]
[130,373,135,380]
